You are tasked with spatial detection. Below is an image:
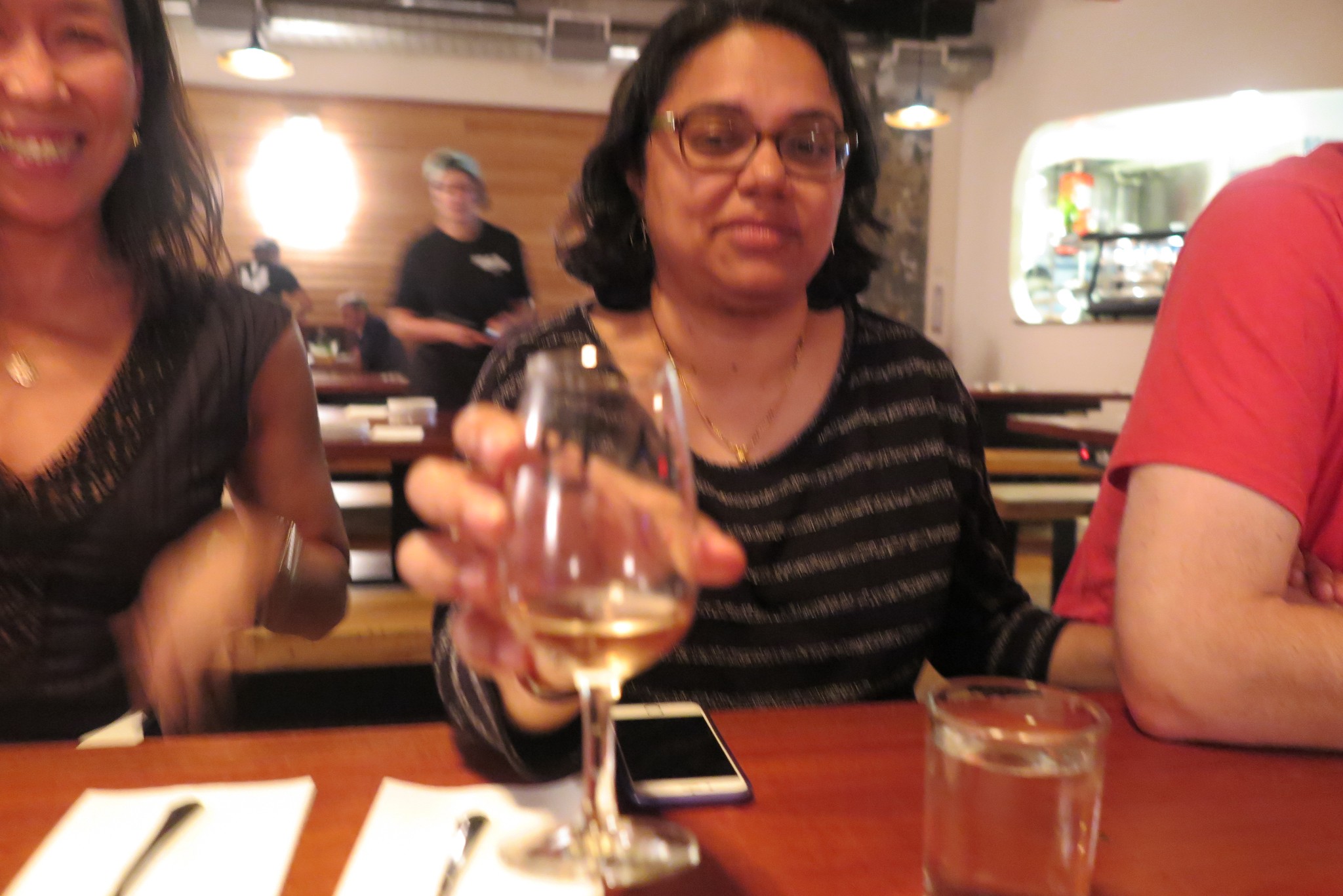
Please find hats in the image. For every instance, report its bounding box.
[420,146,488,201]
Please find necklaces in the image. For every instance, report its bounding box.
[5,352,36,390]
[660,331,804,466]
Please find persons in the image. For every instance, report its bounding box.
[388,148,530,406]
[339,297,403,373]
[225,242,314,321]
[1056,141,1341,751]
[0,0,349,741]
[396,1,1342,781]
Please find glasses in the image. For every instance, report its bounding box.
[649,107,856,180]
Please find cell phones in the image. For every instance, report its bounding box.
[609,702,753,808]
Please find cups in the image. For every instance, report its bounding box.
[921,673,1111,896]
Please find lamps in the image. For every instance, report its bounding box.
[216,0,296,82]
[883,8,951,131]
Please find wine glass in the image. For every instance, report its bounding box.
[498,346,702,890]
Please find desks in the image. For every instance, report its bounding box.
[0,350,1343,896]
